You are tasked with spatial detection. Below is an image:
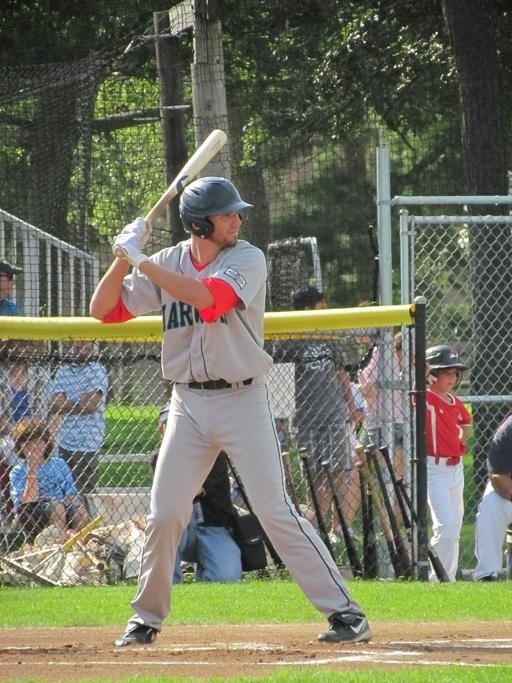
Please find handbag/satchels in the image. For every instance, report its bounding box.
[234,511,270,573]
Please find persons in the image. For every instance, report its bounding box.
[410,344,471,581]
[470,413,512,581]
[90,176,373,644]
[1,257,28,316]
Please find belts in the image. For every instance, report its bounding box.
[426,453,462,466]
[173,373,256,389]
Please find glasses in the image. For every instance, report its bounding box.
[0,274,14,281]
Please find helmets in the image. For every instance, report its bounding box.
[179,174,256,240]
[424,343,467,388]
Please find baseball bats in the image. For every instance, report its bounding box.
[111,128,227,257]
[280,444,448,583]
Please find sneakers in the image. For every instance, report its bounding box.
[113,621,159,647]
[479,576,497,582]
[316,608,376,645]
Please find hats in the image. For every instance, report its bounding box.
[12,418,56,461]
[0,257,24,274]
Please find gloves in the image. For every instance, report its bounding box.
[111,234,154,271]
[123,215,153,240]
